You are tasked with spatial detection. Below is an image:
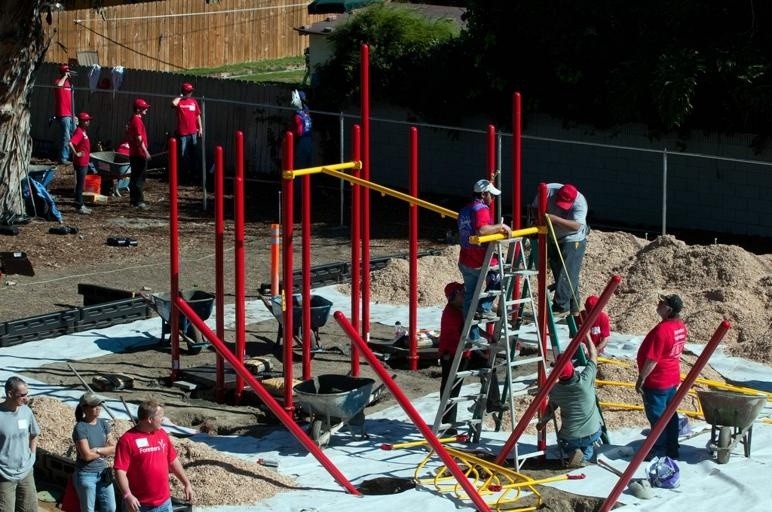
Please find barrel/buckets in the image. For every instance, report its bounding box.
[83,175,102,195]
[392,321,404,340]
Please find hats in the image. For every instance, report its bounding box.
[551,184,577,210]
[134,98,151,108]
[182,82,197,92]
[59,64,71,72]
[474,179,502,195]
[585,296,599,314]
[78,112,94,120]
[79,392,105,407]
[557,353,574,380]
[658,293,683,313]
[444,281,464,299]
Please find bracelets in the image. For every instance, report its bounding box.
[122,492,132,499]
[638,375,645,385]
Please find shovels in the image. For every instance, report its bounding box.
[598,459,655,499]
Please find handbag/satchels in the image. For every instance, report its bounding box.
[100,466,113,488]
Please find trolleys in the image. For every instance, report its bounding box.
[89,146,169,198]
[256,290,343,362]
[140,286,217,356]
[28,159,58,190]
[293,371,400,449]
[695,386,766,464]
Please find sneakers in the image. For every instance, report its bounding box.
[77,205,93,215]
[568,449,587,467]
[475,309,497,320]
[136,199,152,211]
[495,407,509,412]
[571,311,580,316]
[553,310,570,319]
[60,160,72,166]
[468,336,488,345]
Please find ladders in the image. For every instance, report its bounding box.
[432,235,564,472]
[467,236,608,472]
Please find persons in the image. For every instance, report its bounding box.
[113,402,193,512]
[124,99,151,209]
[291,90,312,184]
[72,393,117,512]
[0,377,41,512]
[171,83,202,176]
[531,183,609,467]
[636,294,687,462]
[68,113,93,215]
[440,180,513,437]
[53,64,73,165]
[116,141,131,161]
